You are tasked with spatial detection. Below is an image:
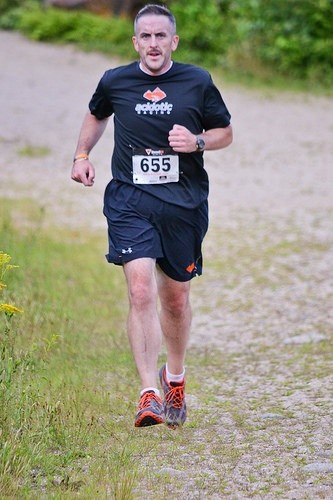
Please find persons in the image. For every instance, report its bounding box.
[71,4,233,430]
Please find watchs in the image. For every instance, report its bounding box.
[196,135,205,153]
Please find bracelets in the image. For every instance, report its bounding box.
[73,154,89,162]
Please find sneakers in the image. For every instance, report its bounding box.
[133,391,166,427]
[159,367,187,430]
